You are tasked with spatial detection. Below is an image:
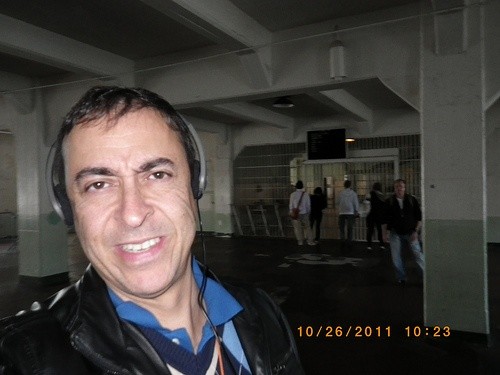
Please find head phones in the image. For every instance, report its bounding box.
[44,111,207,225]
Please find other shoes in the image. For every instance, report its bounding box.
[418,277,423,286]
[399,280,409,289]
[339,239,352,245]
[379,246,386,250]
[298,240,304,245]
[363,246,372,253]
[307,241,316,245]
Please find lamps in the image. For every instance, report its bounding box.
[329,23,347,82]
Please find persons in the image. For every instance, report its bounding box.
[360,182,388,250]
[0,83,305,374]
[381,179,424,286]
[289,179,317,245]
[335,179,359,248]
[308,186,328,244]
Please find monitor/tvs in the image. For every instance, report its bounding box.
[305,127,349,159]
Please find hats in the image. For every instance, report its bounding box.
[296,181,304,189]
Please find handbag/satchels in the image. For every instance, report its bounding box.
[288,208,299,220]
